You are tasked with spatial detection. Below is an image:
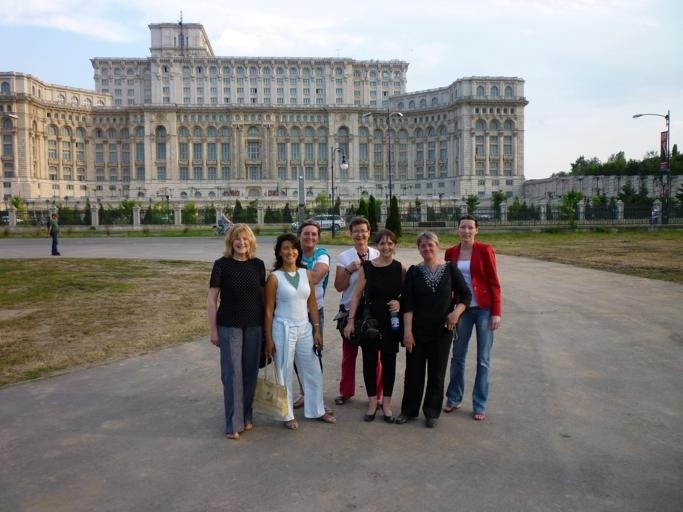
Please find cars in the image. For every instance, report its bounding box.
[291,214,348,232]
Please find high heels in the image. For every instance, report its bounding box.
[363,401,379,422]
[380,404,395,423]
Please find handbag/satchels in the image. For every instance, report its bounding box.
[251,354,289,417]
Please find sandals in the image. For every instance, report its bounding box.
[320,413,337,424]
[293,394,304,408]
[244,422,253,431]
[224,431,240,439]
[324,404,334,416]
[285,418,298,430]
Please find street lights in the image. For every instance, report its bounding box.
[332,147,348,238]
[633,110,671,215]
[362,108,403,206]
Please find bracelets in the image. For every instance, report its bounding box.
[313,323,320,327]
[347,315,354,321]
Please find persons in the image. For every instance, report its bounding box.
[218,212,231,235]
[395,229,473,430]
[442,214,502,421]
[343,229,407,424]
[333,216,384,411]
[47,213,61,256]
[293,221,333,415]
[205,223,267,440]
[264,233,336,430]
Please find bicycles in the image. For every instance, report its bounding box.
[211,223,234,236]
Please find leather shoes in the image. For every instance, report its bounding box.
[396,414,409,424]
[425,417,438,428]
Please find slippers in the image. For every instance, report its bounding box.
[443,404,455,413]
[334,396,348,405]
[473,413,486,421]
